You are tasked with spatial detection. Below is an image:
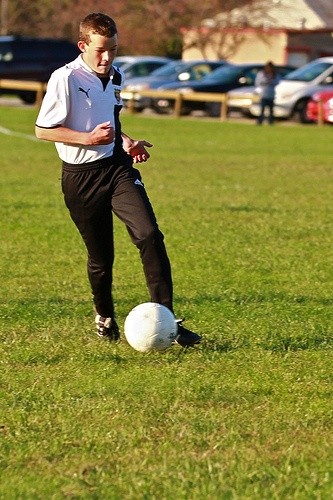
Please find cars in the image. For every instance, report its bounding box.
[222,55,333,122]
[306,81,333,125]
[152,64,300,119]
[121,60,232,116]
[112,55,171,82]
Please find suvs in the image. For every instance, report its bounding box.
[0,35,85,107]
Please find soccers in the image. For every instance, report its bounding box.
[120,301,178,354]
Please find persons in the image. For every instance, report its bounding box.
[254,62,279,125]
[35,12,202,346]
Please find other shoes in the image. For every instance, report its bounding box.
[172,318,203,350]
[255,115,265,125]
[94,313,125,345]
[267,114,276,124]
